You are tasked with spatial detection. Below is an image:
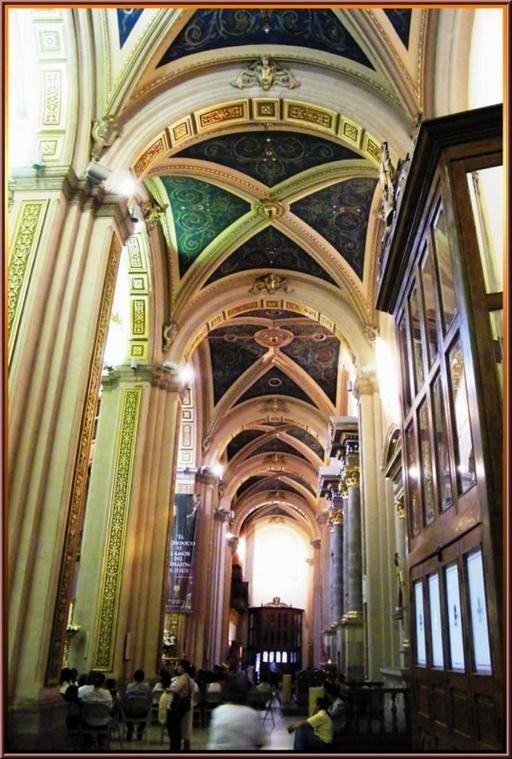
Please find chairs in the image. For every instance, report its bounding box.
[60,673,279,750]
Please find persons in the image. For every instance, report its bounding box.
[58,654,345,750]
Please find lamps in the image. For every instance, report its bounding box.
[86,161,113,185]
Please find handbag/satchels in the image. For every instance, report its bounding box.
[171,695,190,713]
